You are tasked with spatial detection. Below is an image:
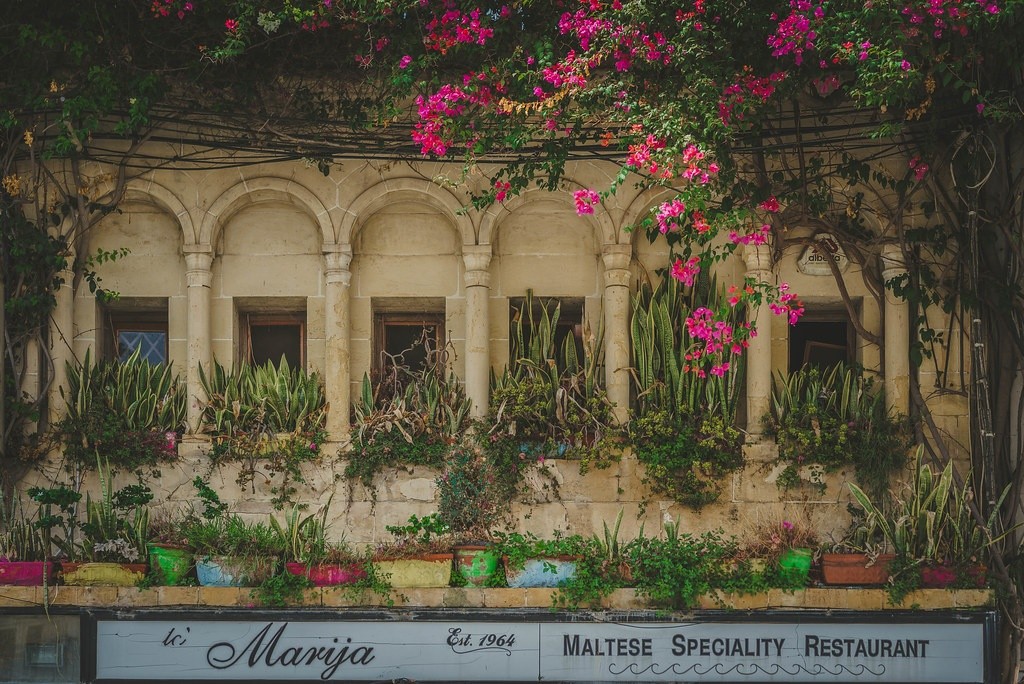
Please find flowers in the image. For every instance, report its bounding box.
[6,421,1024,614]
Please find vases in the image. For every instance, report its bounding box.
[195,553,275,589]
[455,542,498,587]
[505,554,579,589]
[146,541,195,589]
[924,560,987,586]
[6,561,53,588]
[776,547,811,584]
[822,553,984,590]
[59,560,146,591]
[565,432,594,451]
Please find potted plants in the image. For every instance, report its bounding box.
[281,498,358,586]
[365,511,451,588]
